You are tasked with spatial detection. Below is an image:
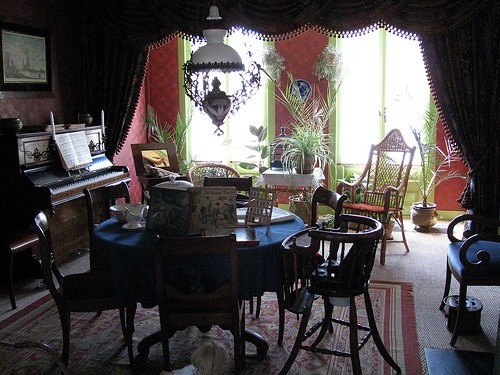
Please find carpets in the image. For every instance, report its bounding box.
[424,348,500,375]
[0,279,423,375]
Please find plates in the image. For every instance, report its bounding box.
[122,221,147,231]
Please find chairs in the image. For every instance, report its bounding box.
[0,130,500,375]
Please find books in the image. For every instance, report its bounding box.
[55,131,93,169]
[146,186,238,239]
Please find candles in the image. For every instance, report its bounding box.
[101,110,106,134]
[50,111,55,140]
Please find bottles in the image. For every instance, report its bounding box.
[79,113,93,126]
[1,117,23,133]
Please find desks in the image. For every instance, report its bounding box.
[255,167,325,227]
[91,207,307,355]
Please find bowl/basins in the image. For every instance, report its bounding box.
[110,203,132,223]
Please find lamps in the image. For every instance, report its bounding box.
[181,0,262,137]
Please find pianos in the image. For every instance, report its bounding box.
[0,122,132,270]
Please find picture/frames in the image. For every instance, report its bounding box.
[0,20,53,92]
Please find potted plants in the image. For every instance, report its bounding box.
[260,67,344,177]
[342,176,372,232]
[374,102,468,234]
[371,195,406,240]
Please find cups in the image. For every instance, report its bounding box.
[124,204,144,228]
